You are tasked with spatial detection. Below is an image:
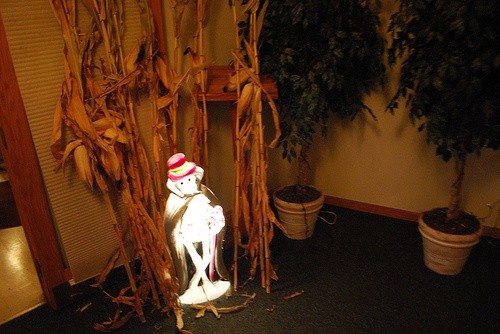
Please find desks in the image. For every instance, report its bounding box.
[189,65,277,238]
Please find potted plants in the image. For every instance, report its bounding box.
[237,0,388,240]
[383,0,500,276]
